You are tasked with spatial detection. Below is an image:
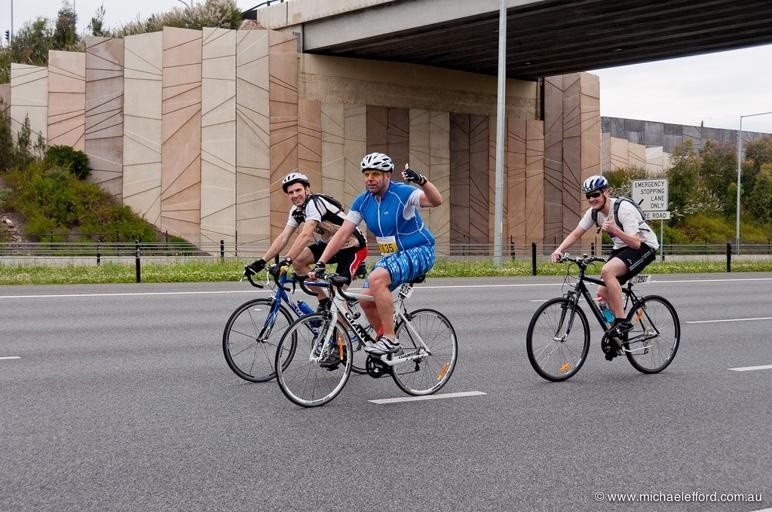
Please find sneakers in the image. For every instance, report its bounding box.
[364,337,403,355]
[319,350,348,369]
[604,350,621,361]
[309,298,332,324]
[606,320,635,338]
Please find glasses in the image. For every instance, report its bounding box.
[586,191,604,199]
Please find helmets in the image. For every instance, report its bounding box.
[580,176,609,193]
[281,172,310,192]
[360,152,395,175]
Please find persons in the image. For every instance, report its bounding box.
[550,175,659,359]
[245,172,369,368]
[308,152,442,355]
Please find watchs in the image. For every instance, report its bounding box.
[283,255,294,265]
[419,175,427,185]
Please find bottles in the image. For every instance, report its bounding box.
[296,299,323,327]
[596,296,615,324]
[352,312,377,340]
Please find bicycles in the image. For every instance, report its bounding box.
[524,252,681,383]
[221,264,458,408]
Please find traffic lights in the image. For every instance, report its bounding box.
[5,30,10,40]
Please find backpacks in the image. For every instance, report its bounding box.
[590,195,646,233]
[302,192,344,219]
